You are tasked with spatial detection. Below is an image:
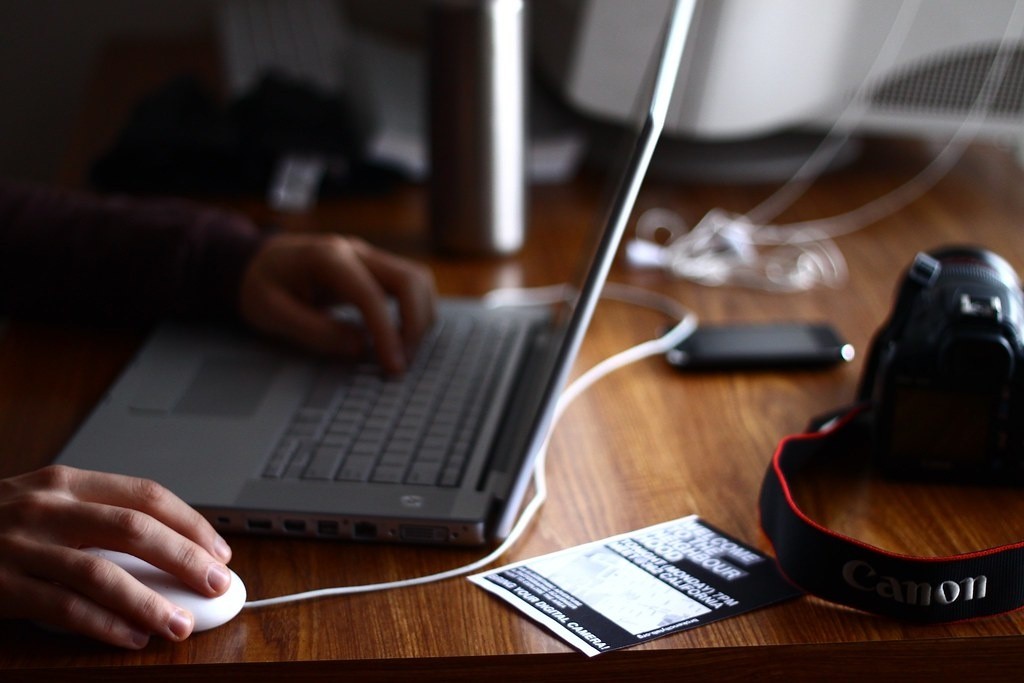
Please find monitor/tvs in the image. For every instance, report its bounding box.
[563,1,1023,180]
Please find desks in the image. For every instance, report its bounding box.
[0,32,1024,683]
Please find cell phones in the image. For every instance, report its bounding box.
[661,319,855,373]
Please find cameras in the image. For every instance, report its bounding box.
[856,244,1023,495]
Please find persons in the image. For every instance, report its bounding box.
[0,177,437,651]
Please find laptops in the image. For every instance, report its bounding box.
[59,0,698,550]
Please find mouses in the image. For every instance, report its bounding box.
[78,548,247,632]
